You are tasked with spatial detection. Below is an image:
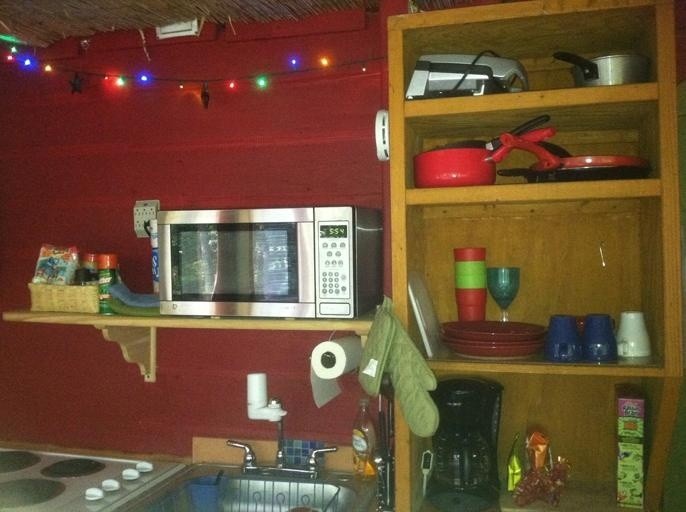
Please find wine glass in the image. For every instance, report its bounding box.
[482,265,520,331]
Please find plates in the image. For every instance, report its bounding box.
[440,320,548,362]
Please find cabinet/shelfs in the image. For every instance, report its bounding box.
[387,0,685,511]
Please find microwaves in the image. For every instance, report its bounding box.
[156,205,384,322]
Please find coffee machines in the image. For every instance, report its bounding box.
[413,375,504,512]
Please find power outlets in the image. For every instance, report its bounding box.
[133,199,161,239]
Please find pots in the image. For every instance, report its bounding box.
[413,48,654,189]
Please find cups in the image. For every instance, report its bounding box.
[452,247,488,324]
[547,310,651,366]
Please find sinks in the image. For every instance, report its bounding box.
[112,461,377,512]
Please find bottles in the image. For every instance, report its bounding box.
[352,398,378,474]
[76,251,118,318]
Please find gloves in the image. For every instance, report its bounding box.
[359,305,399,398]
[390,318,440,439]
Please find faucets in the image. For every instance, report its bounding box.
[267,398,283,451]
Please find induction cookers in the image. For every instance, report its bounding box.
[0,447,186,512]
